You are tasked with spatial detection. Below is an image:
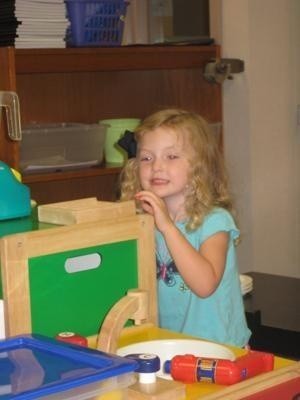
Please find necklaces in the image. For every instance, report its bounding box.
[156,201,183,281]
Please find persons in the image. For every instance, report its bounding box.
[116,107,253,350]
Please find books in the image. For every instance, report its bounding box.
[0,0,70,48]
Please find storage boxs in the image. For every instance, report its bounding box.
[0,333,137,400]
[19,121,111,175]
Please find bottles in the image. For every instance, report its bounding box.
[163,354,247,386]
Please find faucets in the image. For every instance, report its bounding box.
[95,289,151,355]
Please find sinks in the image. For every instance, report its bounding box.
[115,336,238,389]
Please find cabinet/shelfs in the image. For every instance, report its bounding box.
[0,45,222,207]
